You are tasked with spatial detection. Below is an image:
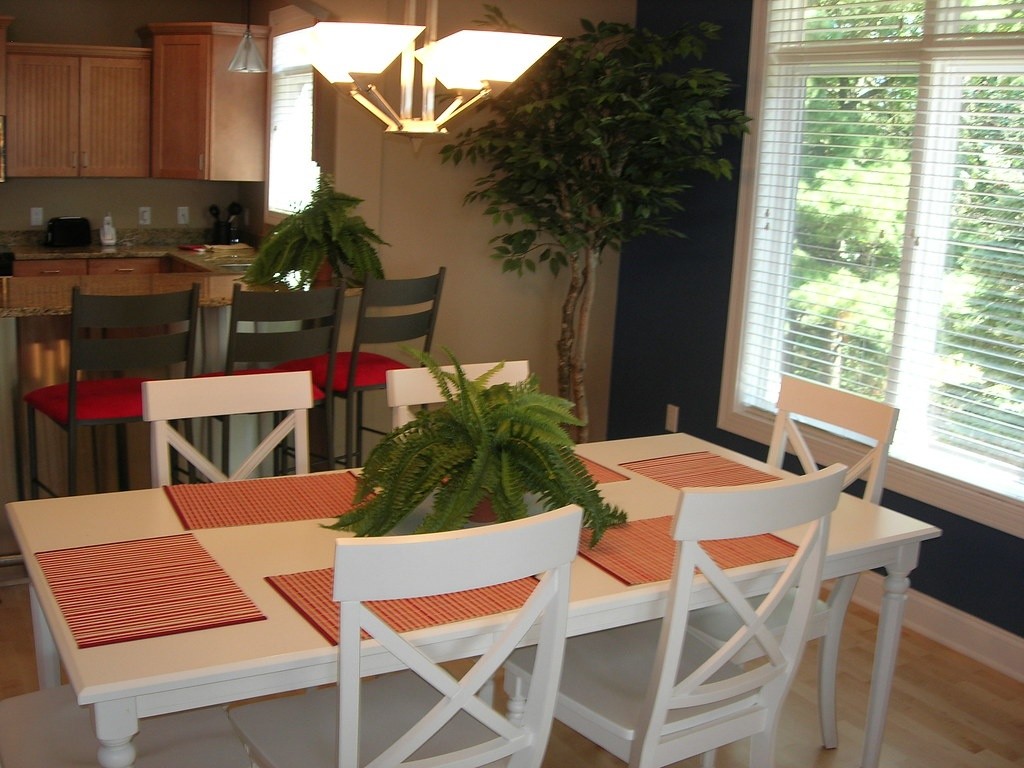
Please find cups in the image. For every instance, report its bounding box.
[214,221,231,246]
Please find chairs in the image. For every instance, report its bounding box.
[273,268,449,477]
[25,281,198,501]
[171,284,346,488]
[0,361,899,767]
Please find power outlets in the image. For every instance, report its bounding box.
[29,207,43,226]
[177,207,190,224]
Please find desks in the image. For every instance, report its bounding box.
[0,276,383,492]
[8,431,943,767]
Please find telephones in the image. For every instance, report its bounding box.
[99,211,116,246]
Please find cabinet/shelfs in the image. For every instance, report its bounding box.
[0,16,271,182]
[12,259,209,275]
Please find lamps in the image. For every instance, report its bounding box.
[270,0,562,140]
[226,1,267,74]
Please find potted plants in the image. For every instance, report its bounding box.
[238,172,393,292]
